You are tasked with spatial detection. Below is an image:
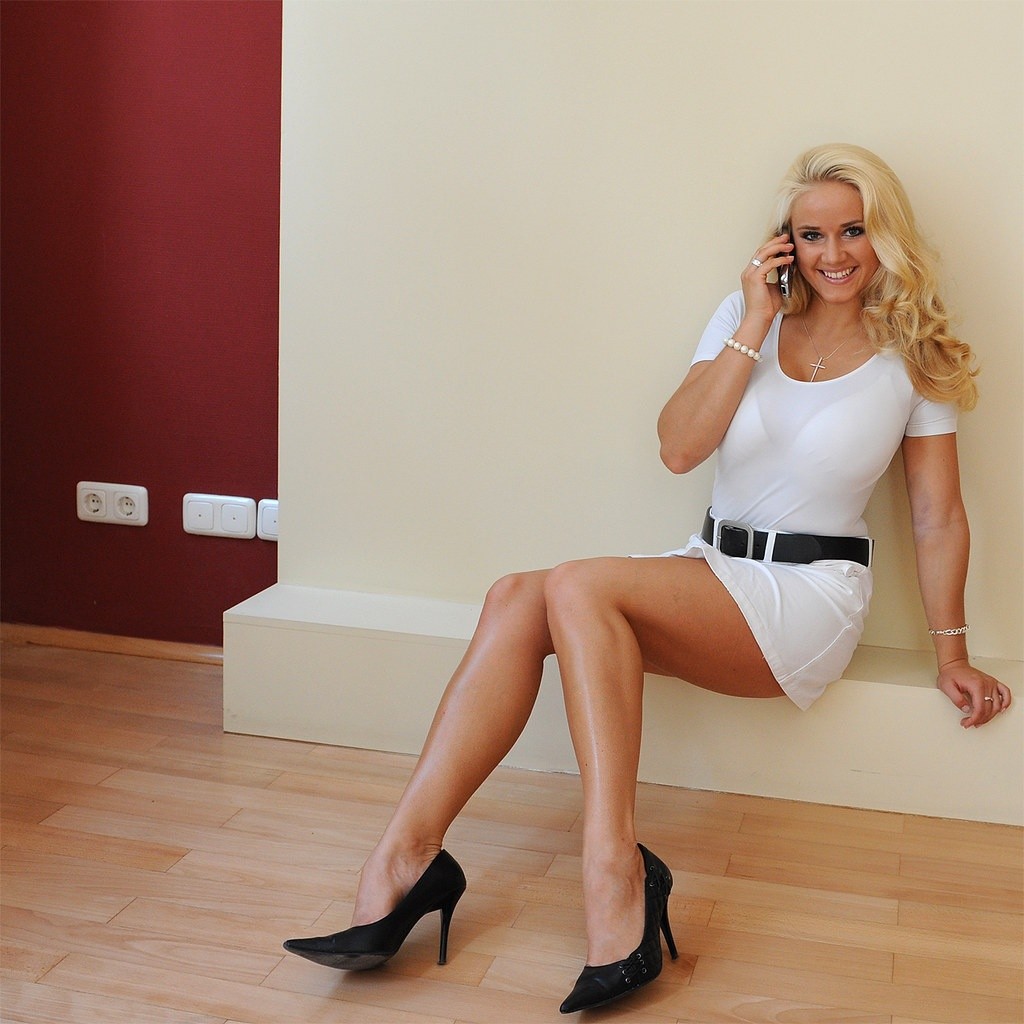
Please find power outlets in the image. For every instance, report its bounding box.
[182,492,257,541]
[75,481,149,528]
[256,500,278,543]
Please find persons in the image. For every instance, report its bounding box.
[282,143,1012,1014]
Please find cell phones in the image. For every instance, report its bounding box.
[779,230,794,299]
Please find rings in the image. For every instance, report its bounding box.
[752,259,762,268]
[984,696,993,702]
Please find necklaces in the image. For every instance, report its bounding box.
[800,314,864,382]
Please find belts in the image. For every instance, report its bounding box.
[702,506,874,570]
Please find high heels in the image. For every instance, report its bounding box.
[560,843,679,1014]
[284,848,467,971]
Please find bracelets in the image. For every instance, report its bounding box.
[928,624,970,636]
[723,338,764,364]
